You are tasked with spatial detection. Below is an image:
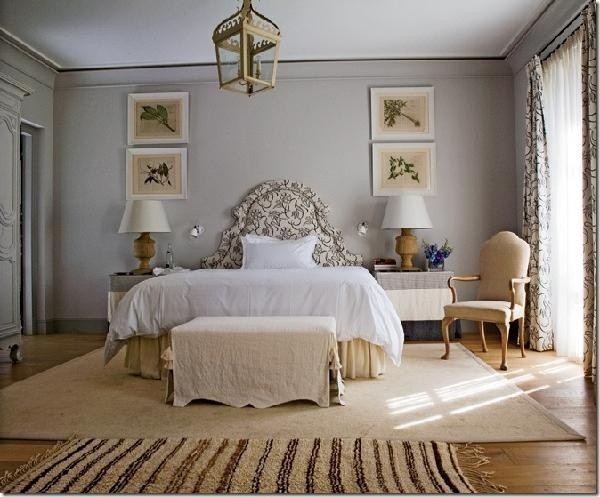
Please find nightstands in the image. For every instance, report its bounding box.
[108,269,190,336]
[372,270,458,341]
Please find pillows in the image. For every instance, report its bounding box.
[241,234,317,269]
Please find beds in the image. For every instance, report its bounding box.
[103,179,405,380]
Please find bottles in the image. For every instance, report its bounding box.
[166,242,173,269]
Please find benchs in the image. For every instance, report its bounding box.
[161,316,345,410]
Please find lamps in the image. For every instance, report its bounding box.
[212,0,282,97]
[381,196,433,272]
[118,200,171,275]
[357,221,369,236]
[190,225,205,238]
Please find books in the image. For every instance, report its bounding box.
[373,258,399,271]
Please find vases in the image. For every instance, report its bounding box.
[426,259,444,271]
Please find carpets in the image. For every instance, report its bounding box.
[0,343,586,443]
[0,433,507,493]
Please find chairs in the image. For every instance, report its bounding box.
[440,231,531,370]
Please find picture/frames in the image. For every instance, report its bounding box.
[372,143,436,197]
[125,148,187,200]
[127,92,189,145]
[371,87,436,141]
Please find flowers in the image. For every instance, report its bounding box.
[422,237,453,268]
